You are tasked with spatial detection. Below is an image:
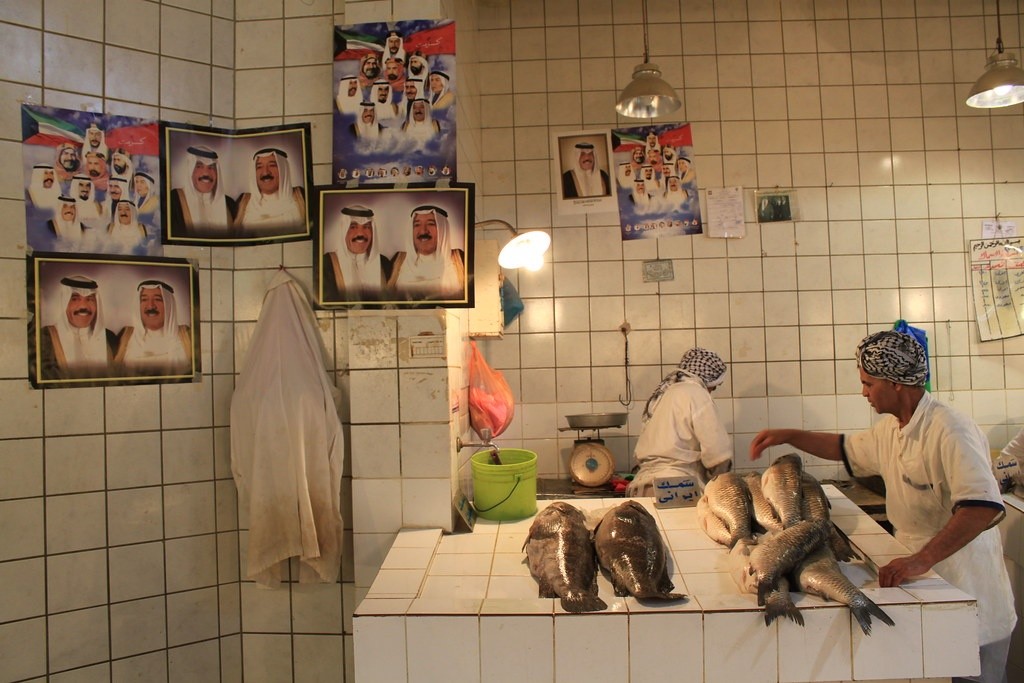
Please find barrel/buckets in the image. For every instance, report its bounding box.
[469,448,538,521]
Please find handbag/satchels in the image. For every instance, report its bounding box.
[471,340,513,440]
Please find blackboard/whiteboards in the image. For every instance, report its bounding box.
[969,236,1024,343]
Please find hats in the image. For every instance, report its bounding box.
[855,330,928,387]
[676,348,727,387]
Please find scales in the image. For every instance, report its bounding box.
[557,412,629,487]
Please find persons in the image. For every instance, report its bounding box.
[170,143,239,240]
[389,203,467,300]
[616,130,696,211]
[115,280,193,378]
[627,347,734,497]
[335,27,454,180]
[42,274,119,379]
[26,121,162,252]
[323,204,393,300]
[235,147,307,235]
[747,329,1018,681]
[561,141,611,200]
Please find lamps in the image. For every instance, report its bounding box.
[965,1,1024,108]
[474,219,551,271]
[615,0,682,118]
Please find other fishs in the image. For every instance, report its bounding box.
[695,451,897,636]
[521,501,608,614]
[593,500,689,602]
